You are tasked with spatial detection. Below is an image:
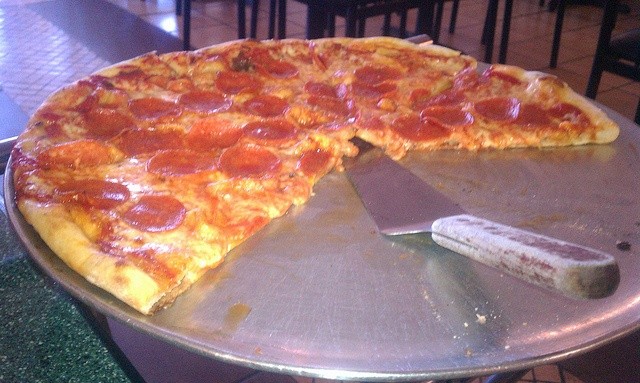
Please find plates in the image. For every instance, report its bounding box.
[5,70,635,380]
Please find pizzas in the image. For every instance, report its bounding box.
[9,36,620,314]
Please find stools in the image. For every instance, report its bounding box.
[303,1,434,43]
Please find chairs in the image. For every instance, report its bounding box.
[585,1,640,126]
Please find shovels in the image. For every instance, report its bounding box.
[342,136,621,301]
